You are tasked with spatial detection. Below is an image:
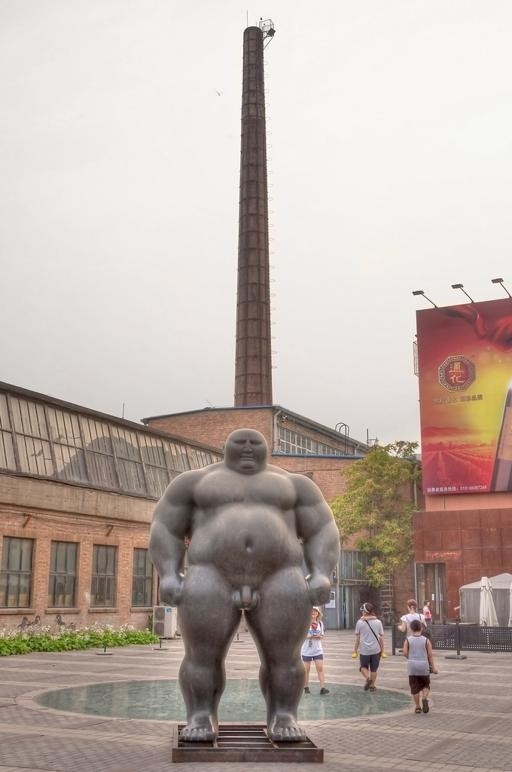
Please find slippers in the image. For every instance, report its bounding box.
[352,652,389,658]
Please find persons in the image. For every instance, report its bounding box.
[422,599,432,622]
[302,605,329,694]
[403,619,439,713]
[398,599,427,638]
[148,428,341,745]
[351,602,388,691]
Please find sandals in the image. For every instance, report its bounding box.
[415,707,422,713]
[422,698,429,713]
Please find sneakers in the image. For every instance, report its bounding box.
[304,686,330,695]
[364,678,376,692]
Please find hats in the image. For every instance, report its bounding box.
[360,603,371,614]
[312,606,323,618]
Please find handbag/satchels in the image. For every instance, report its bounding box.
[421,624,433,644]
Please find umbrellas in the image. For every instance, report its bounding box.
[479,575,498,647]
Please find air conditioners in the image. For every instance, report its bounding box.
[151,605,177,638]
